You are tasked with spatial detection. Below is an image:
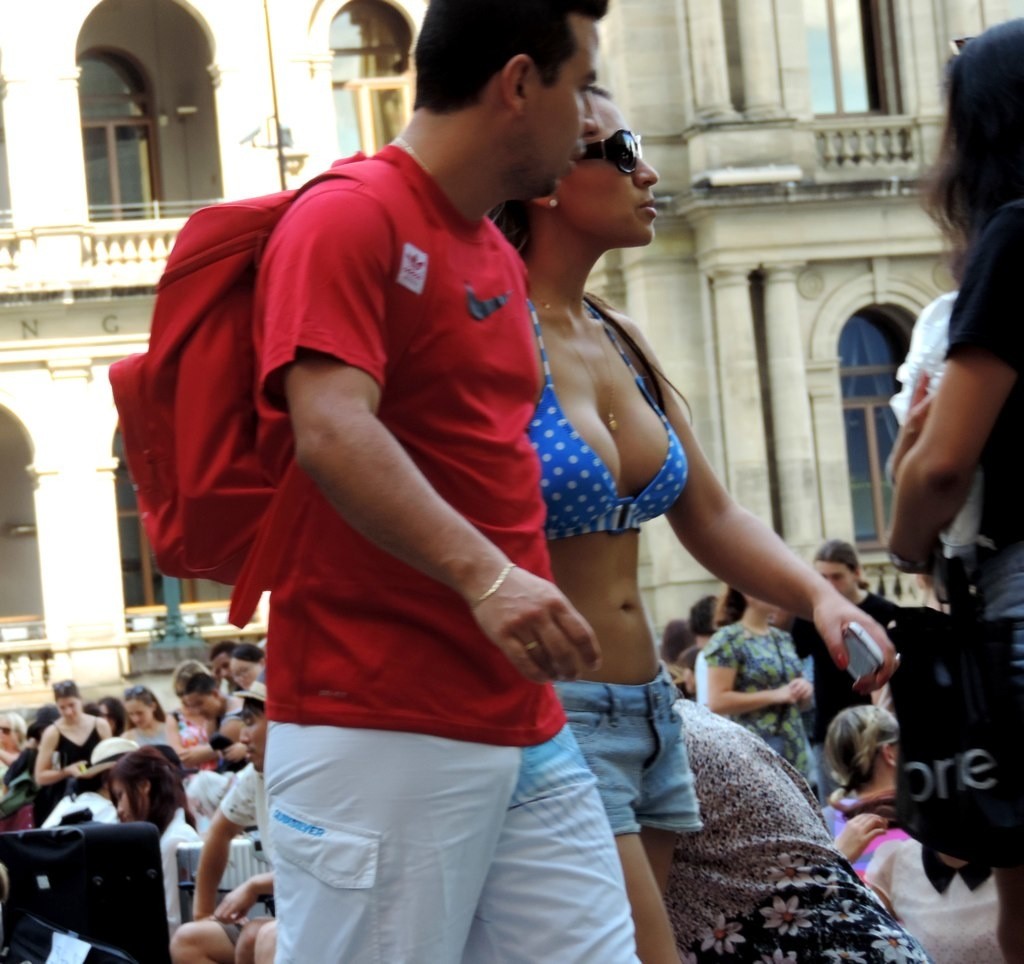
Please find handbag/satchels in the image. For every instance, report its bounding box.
[888,556,1018,869]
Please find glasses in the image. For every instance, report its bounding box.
[0,726,11,735]
[52,681,75,689]
[580,129,642,173]
[124,685,148,696]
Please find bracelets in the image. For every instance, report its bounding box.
[470,562,516,612]
[885,540,928,568]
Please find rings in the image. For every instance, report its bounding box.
[523,641,539,650]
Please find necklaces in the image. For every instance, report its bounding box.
[523,277,617,430]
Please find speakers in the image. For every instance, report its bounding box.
[0,821,174,964]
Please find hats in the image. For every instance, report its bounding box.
[81,738,140,779]
[233,665,265,705]
[154,745,198,775]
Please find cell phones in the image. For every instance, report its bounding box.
[838,620,885,682]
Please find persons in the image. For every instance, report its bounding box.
[0,1,1024,964]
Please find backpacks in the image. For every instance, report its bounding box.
[109,162,434,628]
